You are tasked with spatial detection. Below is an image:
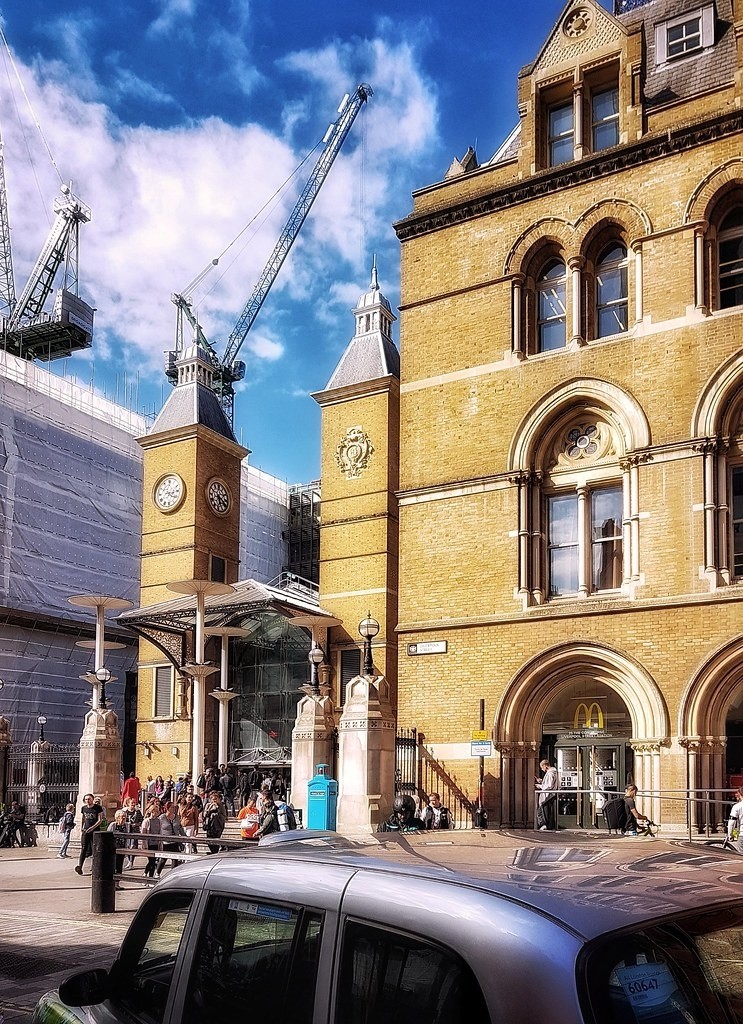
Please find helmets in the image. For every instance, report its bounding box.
[392,795,416,824]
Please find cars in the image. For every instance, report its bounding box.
[28,825,742,1024]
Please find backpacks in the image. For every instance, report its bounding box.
[276,803,298,832]
[196,774,205,788]
[58,812,74,833]
[601,798,627,829]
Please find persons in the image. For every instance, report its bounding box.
[536,760,558,831]
[107,763,296,890]
[8,801,25,848]
[74,794,106,875]
[621,785,647,836]
[728,787,743,857]
[58,803,76,859]
[376,793,455,833]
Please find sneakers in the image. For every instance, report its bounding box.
[62,853,71,859]
[57,853,65,859]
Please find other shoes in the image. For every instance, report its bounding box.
[74,865,83,875]
[125,861,134,869]
[115,882,125,890]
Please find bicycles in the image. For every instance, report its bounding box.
[635,816,661,837]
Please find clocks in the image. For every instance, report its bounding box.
[155,471,190,519]
[202,476,237,520]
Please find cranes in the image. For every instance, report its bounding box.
[160,82,375,432]
[0,107,99,364]
